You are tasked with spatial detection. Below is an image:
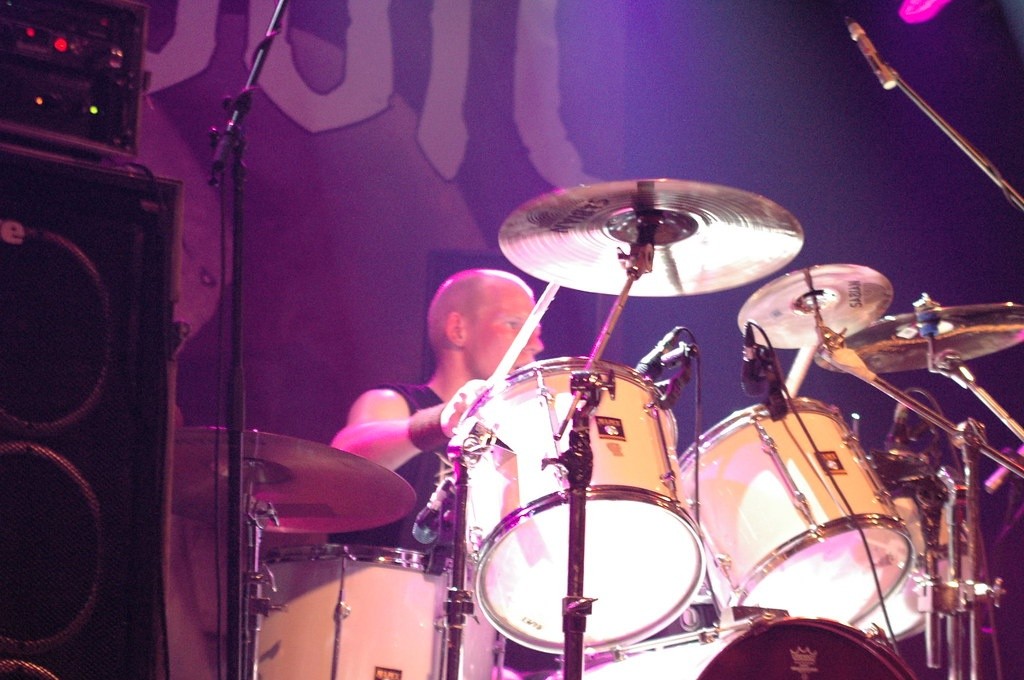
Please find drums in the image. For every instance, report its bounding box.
[559,595,792,675]
[677,396,916,628]
[255,544,498,680]
[456,356,708,656]
[840,483,982,644]
[545,618,920,680]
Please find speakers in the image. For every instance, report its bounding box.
[0,139,180,680]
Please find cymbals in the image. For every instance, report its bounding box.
[737,264,894,350]
[498,178,806,298]
[171,425,418,535]
[813,302,1024,373]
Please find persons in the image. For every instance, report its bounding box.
[330,270,544,576]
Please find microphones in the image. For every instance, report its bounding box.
[884,403,910,452]
[845,17,897,89]
[740,324,770,398]
[634,326,681,380]
[982,444,1024,495]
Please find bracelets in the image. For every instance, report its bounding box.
[409,405,451,452]
